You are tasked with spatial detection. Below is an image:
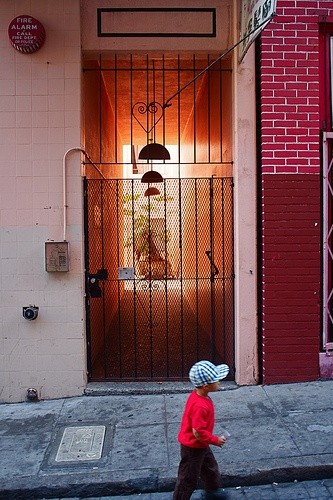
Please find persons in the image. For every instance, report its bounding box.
[172,360,230,500]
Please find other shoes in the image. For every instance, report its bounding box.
[202,490,229,500]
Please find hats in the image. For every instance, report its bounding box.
[189,360,230,387]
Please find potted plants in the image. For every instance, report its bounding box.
[122,193,178,278]
